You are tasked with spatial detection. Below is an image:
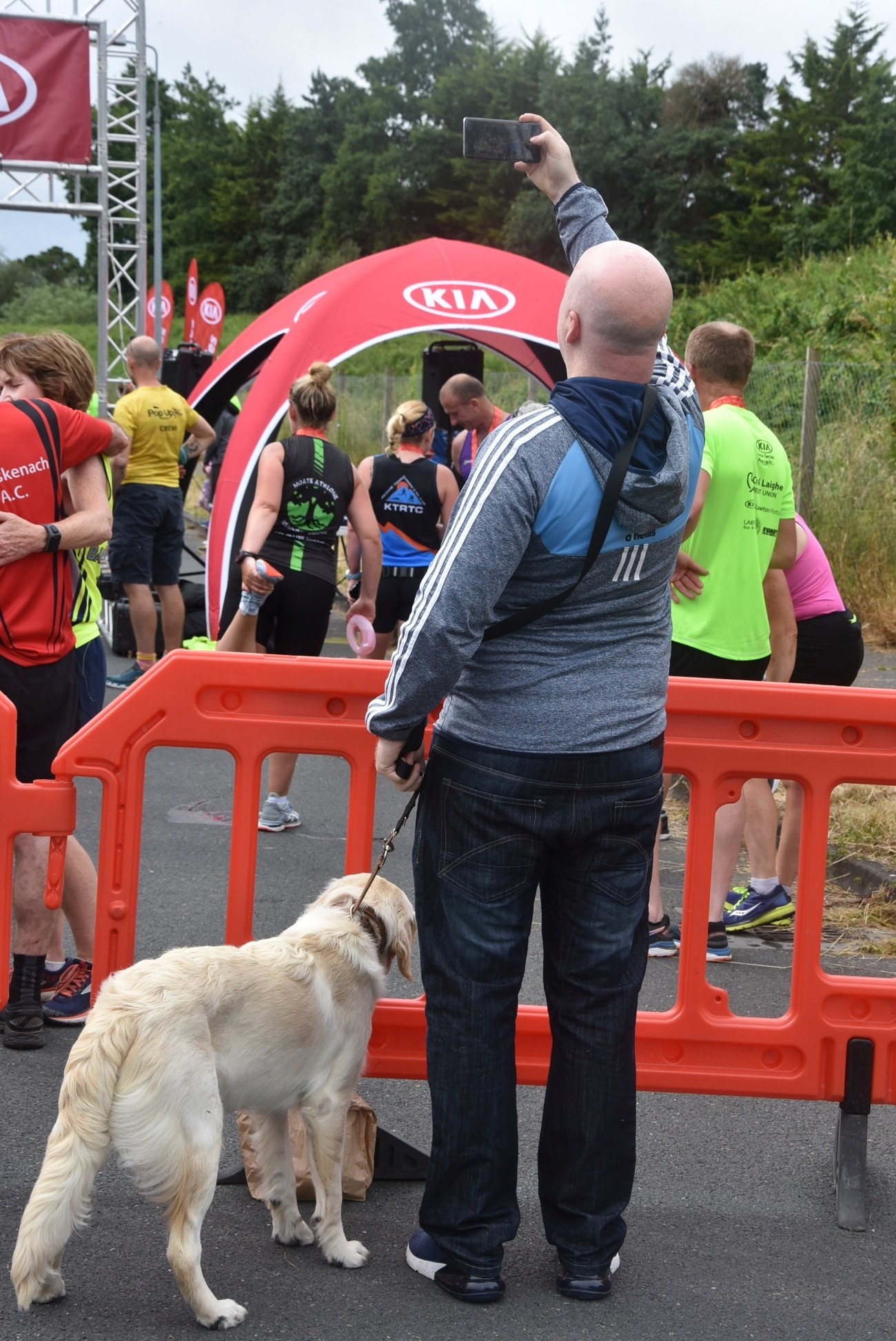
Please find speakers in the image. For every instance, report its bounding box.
[422,339,484,432]
[162,342,214,399]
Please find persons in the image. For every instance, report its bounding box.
[438,373,513,486]
[365,113,705,1304]
[645,319,865,962]
[0,331,241,1051]
[345,399,459,660]
[207,360,383,833]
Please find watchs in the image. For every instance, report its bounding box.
[234,549,260,564]
[40,523,62,553]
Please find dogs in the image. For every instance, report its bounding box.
[7,873,416,1329]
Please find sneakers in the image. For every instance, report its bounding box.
[242,559,285,616]
[40,957,72,1000]
[723,885,792,925]
[258,800,301,832]
[0,1000,45,1050]
[556,1252,620,1299]
[723,884,796,931]
[406,1231,507,1305]
[648,914,677,957]
[42,958,93,1026]
[105,662,147,689]
[670,921,732,961]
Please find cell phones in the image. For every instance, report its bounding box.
[463,117,542,163]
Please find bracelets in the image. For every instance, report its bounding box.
[345,568,362,580]
